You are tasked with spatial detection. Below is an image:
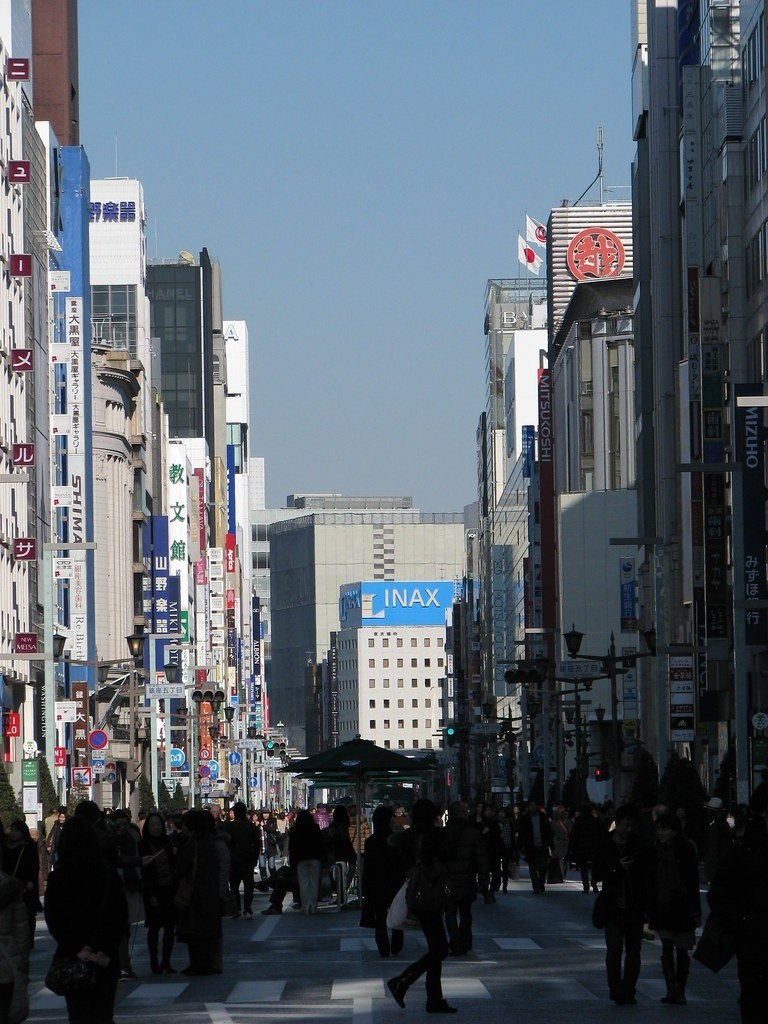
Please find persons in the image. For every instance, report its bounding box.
[0,821,40,1024]
[42,769,768,1024]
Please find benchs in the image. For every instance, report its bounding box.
[293,860,349,915]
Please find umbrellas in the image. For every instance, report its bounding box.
[277,737,440,908]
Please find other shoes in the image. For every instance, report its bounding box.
[533,887,545,895]
[117,970,137,981]
[261,905,282,915]
[254,880,269,892]
[481,891,496,904]
[607,986,641,1006]
[584,881,598,894]
[231,910,253,920]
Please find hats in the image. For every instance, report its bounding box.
[702,797,726,811]
[229,802,247,813]
[260,808,271,814]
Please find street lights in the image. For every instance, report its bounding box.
[50,631,145,818]
[177,701,293,809]
[93,659,178,823]
[456,620,656,818]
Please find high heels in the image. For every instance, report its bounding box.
[151,959,177,974]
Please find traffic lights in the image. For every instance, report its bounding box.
[191,689,229,701]
[266,741,287,750]
[448,724,455,745]
[595,768,602,781]
[503,667,545,684]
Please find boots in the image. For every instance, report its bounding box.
[426,981,457,1013]
[387,958,422,1008]
[502,881,509,894]
[260,867,276,881]
[659,955,690,1006]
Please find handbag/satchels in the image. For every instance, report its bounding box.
[386,878,411,931]
[592,890,611,929]
[164,881,195,909]
[270,832,284,845]
[45,951,107,997]
[7,967,31,1022]
[547,858,564,883]
[359,898,375,928]
[508,862,519,880]
[406,879,456,912]
